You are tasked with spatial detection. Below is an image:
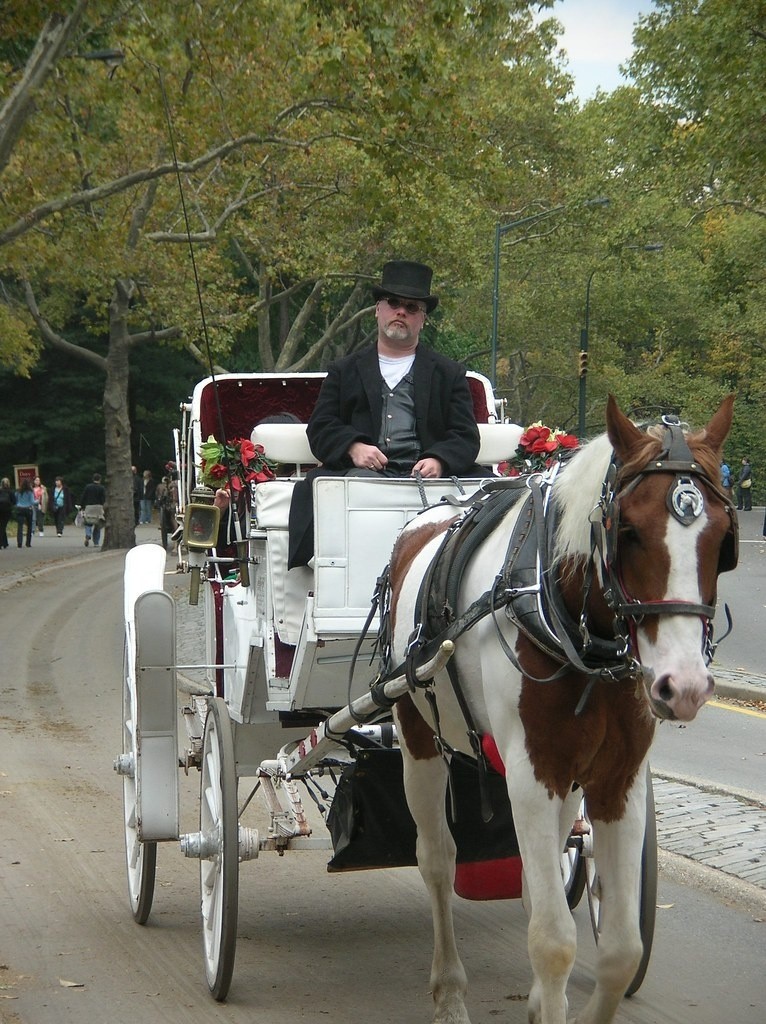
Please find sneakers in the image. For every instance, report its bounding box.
[38,532,43,536]
[57,533,61,537]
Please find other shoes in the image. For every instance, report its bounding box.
[94,541,99,546]
[84,539,89,547]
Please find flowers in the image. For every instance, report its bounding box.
[201,436,276,515]
[497,421,584,478]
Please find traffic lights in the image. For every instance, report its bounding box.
[579,349,588,378]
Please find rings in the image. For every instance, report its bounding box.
[369,465,375,469]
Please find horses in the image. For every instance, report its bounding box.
[378,393,743,1024]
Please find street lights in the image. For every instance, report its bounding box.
[580,245,664,440]
[492,198,611,401]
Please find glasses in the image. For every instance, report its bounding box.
[380,297,425,313]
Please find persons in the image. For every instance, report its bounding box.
[288,260,499,571]
[736,458,753,510]
[78,474,106,547]
[50,477,73,537]
[762,506,766,541]
[0,478,18,549]
[131,466,168,530]
[209,412,318,578]
[719,458,732,489]
[31,476,49,536]
[15,477,40,548]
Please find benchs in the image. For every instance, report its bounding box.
[250,423,525,529]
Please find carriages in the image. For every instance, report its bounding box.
[111,370,734,1024]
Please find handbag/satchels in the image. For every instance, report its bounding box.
[740,478,752,488]
[73,510,83,528]
[50,501,58,516]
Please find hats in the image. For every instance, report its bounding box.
[366,261,439,315]
